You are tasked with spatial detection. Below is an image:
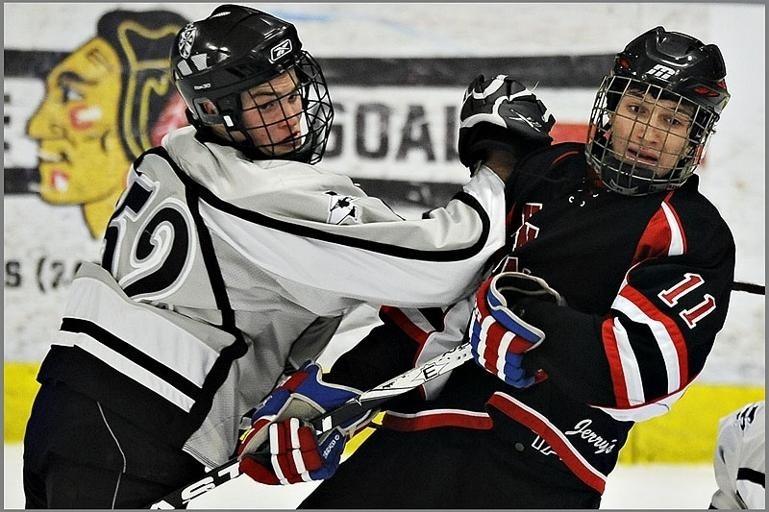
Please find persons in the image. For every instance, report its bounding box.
[709,402,767,509]
[25,11,189,242]
[23,3,554,509]
[235,25,731,509]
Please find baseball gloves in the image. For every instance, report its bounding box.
[459,69,552,156]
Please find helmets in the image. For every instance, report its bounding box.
[169,4,334,165]
[583,25,732,196]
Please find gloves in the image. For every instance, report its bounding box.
[468,267,566,390]
[458,71,557,170]
[234,358,373,486]
